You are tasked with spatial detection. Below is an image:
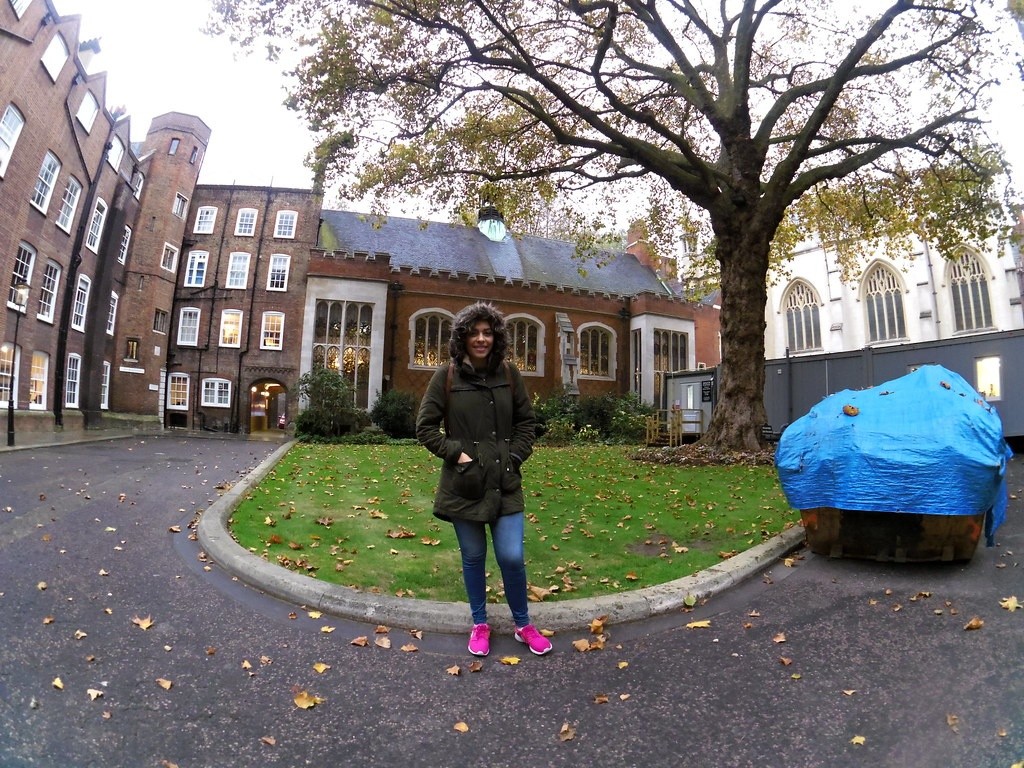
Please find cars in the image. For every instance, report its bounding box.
[278,414,285,428]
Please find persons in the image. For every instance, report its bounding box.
[416,302,552,656]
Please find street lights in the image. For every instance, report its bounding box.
[7,275,33,447]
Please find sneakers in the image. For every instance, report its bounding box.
[515,624,552,655]
[467,624,489,656]
